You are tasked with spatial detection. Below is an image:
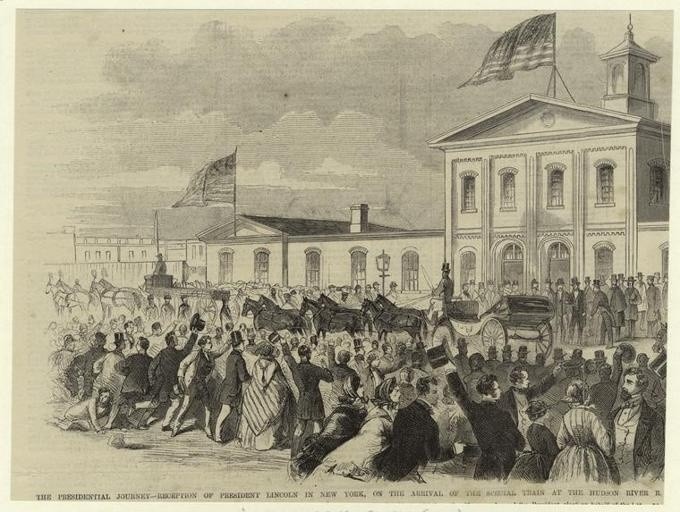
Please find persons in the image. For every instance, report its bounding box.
[45,251,669,484]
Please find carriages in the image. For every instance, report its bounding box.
[241,292,556,359]
[45,274,241,334]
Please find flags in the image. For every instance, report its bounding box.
[169,151,235,209]
[455,12,556,90]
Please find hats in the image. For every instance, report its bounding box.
[101,253,668,364]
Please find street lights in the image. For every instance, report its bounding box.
[376,248,391,297]
